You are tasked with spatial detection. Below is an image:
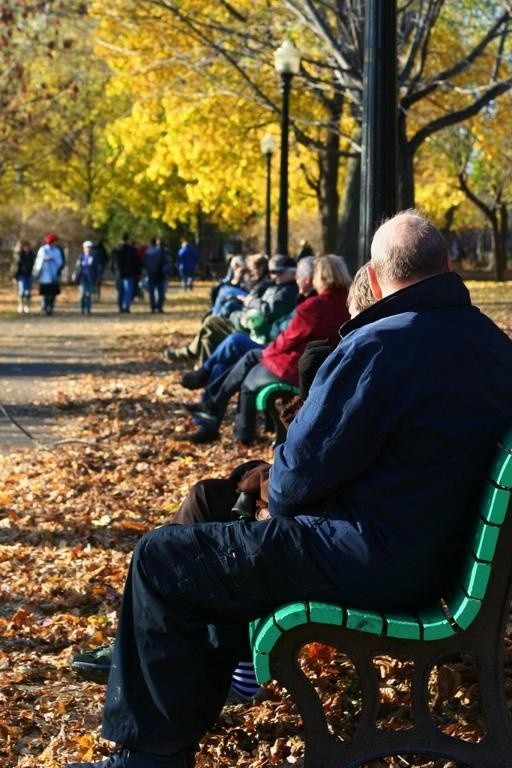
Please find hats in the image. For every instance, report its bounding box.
[82,240,94,248]
[44,233,56,245]
[266,253,297,273]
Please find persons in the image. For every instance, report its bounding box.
[11,233,65,314]
[65,209,511,768]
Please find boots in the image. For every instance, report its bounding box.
[17,295,32,314]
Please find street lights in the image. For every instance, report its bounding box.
[268,37,308,263]
[259,128,278,256]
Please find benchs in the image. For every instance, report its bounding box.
[240,428,511,768]
[251,382,304,444]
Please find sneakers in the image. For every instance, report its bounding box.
[179,401,221,432]
[162,347,197,368]
[180,366,211,390]
[63,746,195,768]
[68,640,113,685]
[171,425,222,444]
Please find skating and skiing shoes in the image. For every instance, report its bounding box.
[223,684,267,707]
